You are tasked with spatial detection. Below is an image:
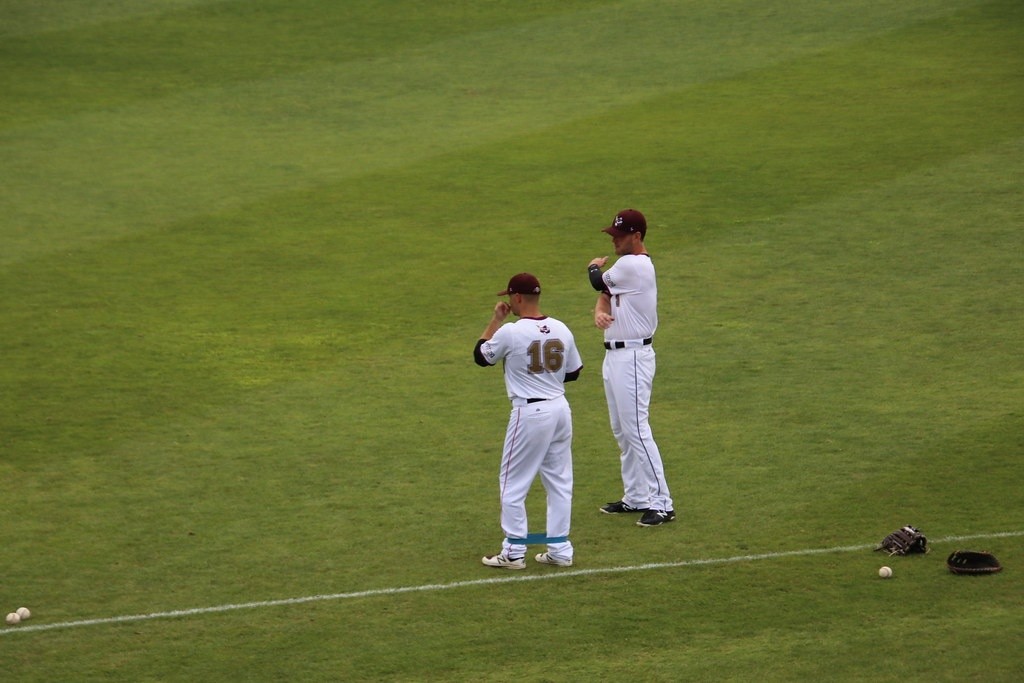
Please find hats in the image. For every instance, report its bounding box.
[601,209,647,237]
[497,273,541,296]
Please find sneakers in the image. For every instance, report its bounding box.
[481,552,527,570]
[636,509,676,527]
[535,552,573,567]
[599,500,649,515]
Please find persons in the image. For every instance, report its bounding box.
[587,209,676,526]
[473,273,583,569]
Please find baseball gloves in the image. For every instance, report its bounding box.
[873,523,931,557]
[947,548,1004,576]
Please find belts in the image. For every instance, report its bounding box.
[527,398,546,403]
[603,337,652,349]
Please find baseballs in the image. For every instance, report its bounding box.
[879,566,893,577]
[6,607,31,625]
